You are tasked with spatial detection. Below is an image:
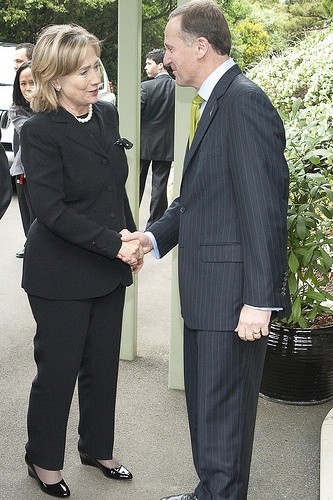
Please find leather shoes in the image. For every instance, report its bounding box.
[160,492,198,500]
[16,249,25,258]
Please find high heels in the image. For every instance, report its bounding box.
[24,452,71,498]
[79,450,134,480]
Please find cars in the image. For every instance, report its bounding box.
[0,40,117,168]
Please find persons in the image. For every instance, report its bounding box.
[116,1,293,500]
[140,48,175,231]
[0,43,36,258]
[20,25,144,498]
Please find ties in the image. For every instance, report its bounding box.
[189,94,206,151]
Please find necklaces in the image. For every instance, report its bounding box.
[69,103,93,123]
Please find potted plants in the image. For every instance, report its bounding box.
[258,99,333,406]
